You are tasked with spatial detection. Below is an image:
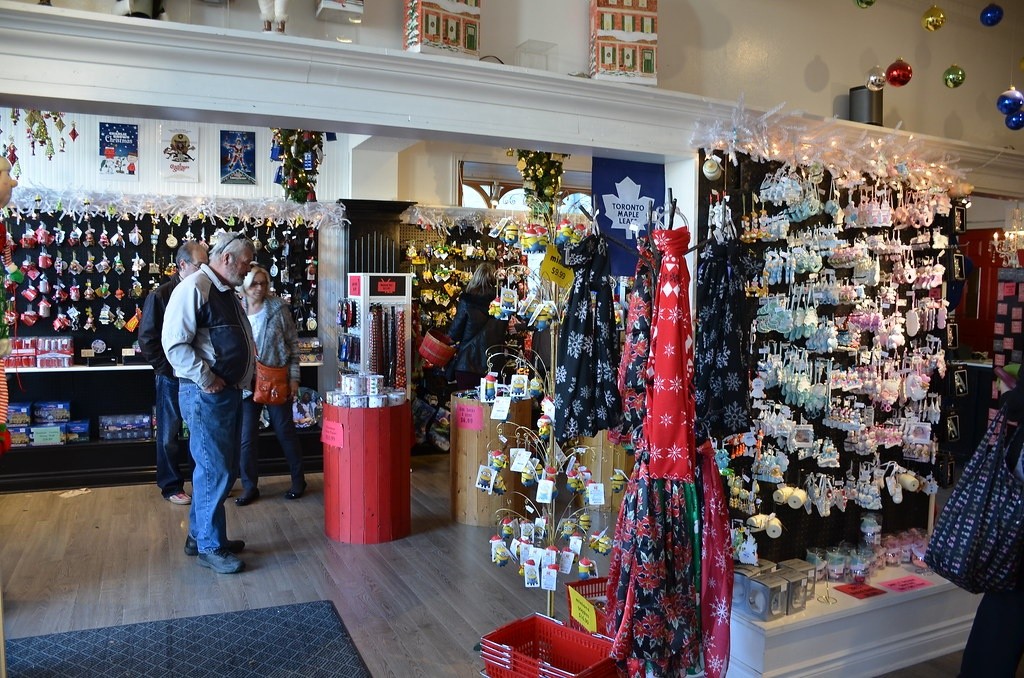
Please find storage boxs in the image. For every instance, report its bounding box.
[4,402,152,447]
[726,555,817,622]
[404,0,481,60]
[588,0,658,87]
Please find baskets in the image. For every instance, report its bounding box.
[478,577,621,678]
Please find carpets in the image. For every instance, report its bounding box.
[4,600,373,678]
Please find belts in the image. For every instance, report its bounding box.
[368,307,406,389]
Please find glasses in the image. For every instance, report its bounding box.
[220,233,252,253]
[188,262,201,271]
[250,280,269,288]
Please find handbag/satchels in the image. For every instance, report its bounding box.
[922,400,1024,594]
[441,361,457,385]
[253,361,288,405]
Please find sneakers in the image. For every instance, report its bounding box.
[235,490,259,506]
[195,549,245,574]
[286,483,307,499]
[184,535,244,556]
[167,493,192,505]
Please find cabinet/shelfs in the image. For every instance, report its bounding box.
[0,359,325,494]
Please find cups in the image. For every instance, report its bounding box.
[703,161,721,182]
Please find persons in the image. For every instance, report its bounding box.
[449,262,506,393]
[138,242,208,504]
[161,232,255,574]
[959,361,1023,678]
[234,261,306,507]
